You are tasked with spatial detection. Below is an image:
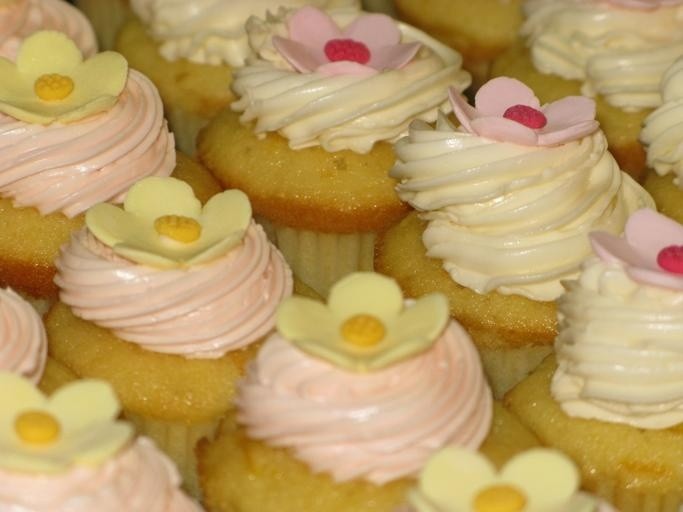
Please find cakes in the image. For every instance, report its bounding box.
[0,0,683,512]
